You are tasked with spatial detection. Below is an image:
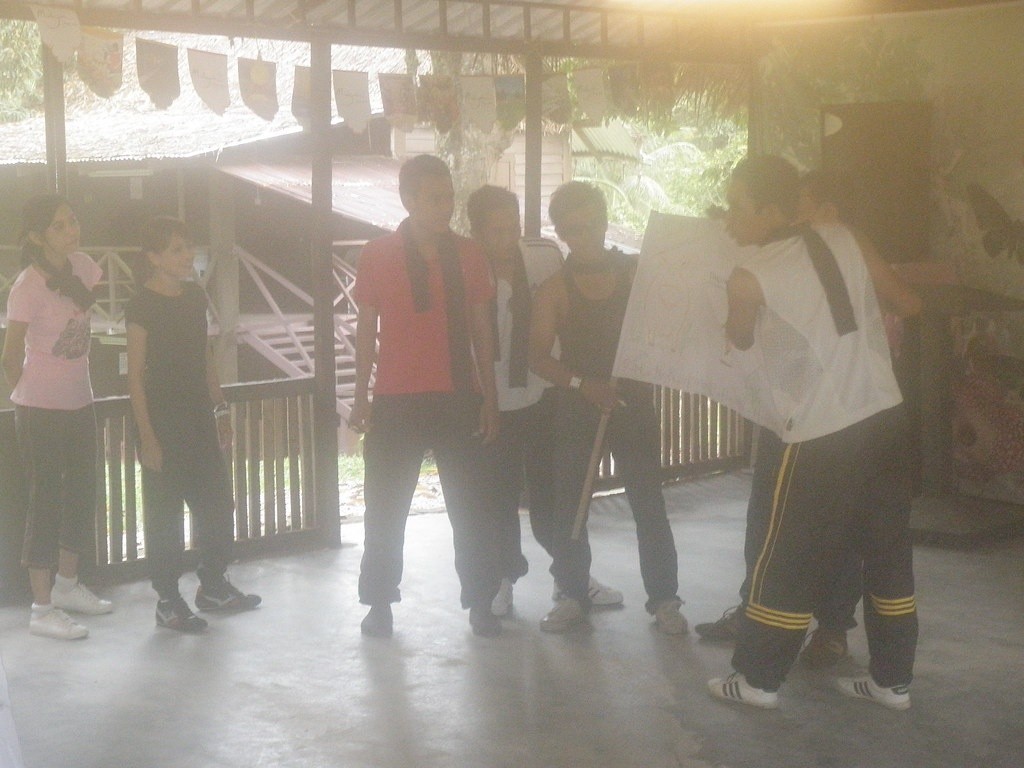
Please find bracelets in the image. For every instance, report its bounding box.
[571,371,581,392]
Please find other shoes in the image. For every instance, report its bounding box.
[539,597,592,634]
[470,607,501,638]
[798,626,847,666]
[696,606,742,641]
[655,607,688,635]
[361,603,393,635]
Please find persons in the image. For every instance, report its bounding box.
[347,153,513,638]
[463,187,622,617]
[125,216,261,631]
[0,194,113,640]
[702,152,921,711]
[527,182,687,634]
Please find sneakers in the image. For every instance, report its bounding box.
[708,672,771,706]
[195,580,261,612]
[552,577,624,605]
[155,597,208,632]
[836,669,912,710]
[491,579,514,616]
[29,609,89,639]
[50,581,113,614]
